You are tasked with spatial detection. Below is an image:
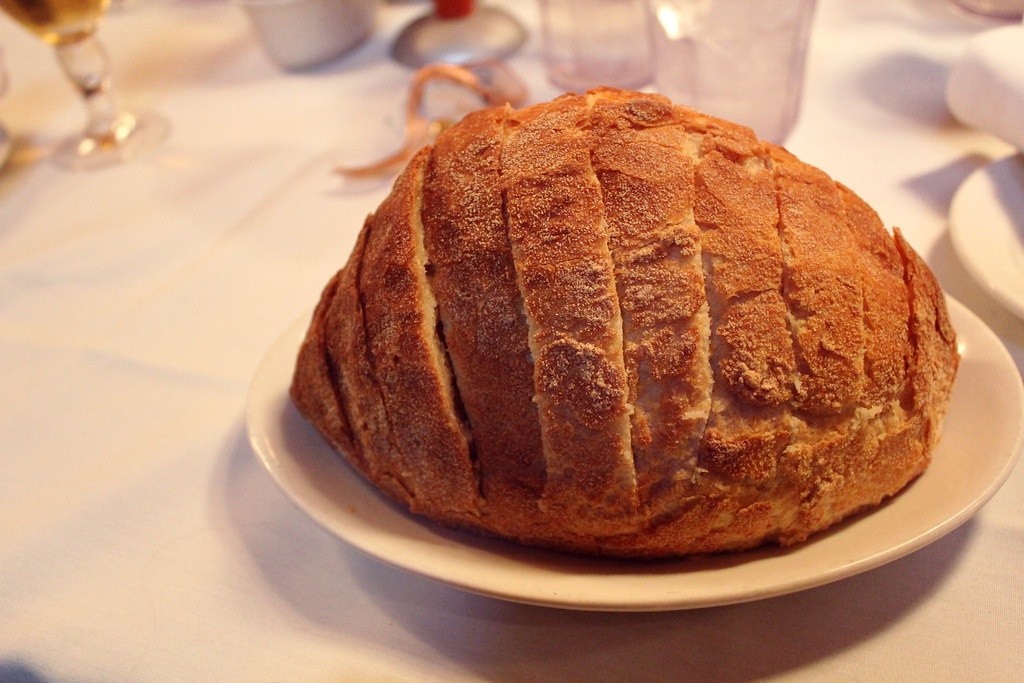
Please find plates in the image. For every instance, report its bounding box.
[242,290,1024,612]
[947,151,1024,318]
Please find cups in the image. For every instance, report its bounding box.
[536,0,656,96]
[236,0,379,69]
[644,0,816,145]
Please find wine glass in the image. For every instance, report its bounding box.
[0,0,180,173]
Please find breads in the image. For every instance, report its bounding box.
[290,87,960,553]
